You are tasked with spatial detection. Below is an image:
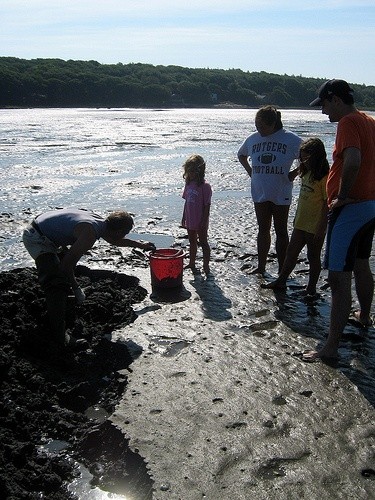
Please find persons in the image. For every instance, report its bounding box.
[22,211,156,346]
[302,80,375,363]
[261,138,331,300]
[182,156,213,275]
[238,106,309,277]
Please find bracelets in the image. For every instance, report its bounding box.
[335,194,346,200]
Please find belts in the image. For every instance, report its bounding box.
[30,220,59,249]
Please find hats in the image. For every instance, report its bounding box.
[309,78,353,107]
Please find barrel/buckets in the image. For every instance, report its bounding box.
[149,249,183,287]
